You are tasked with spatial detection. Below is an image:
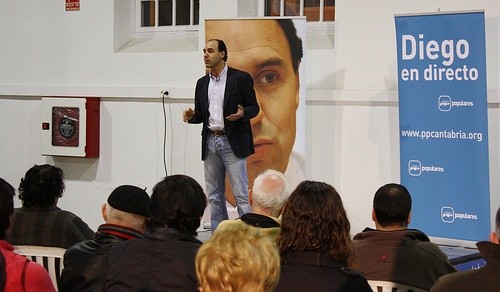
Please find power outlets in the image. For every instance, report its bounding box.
[160,88,170,99]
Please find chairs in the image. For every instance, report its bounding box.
[12,246,67,292]
[367,280,422,292]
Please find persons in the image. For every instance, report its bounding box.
[213,169,290,236]
[5,164,95,280]
[273,180,373,292]
[195,219,281,292]
[348,183,500,292]
[88,174,208,292]
[58,185,151,292]
[205,19,303,209]
[0,177,57,292]
[183,39,260,236]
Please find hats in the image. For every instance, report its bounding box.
[108,185,150,218]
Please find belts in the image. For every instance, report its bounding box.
[208,129,226,136]
